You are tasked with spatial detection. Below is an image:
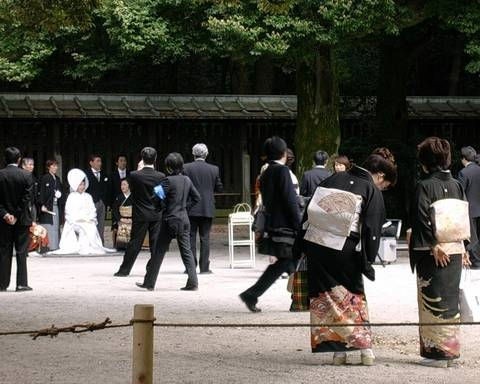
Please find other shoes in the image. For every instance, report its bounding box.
[180,286,198,290]
[333,353,346,365]
[136,281,154,291]
[421,358,457,368]
[361,349,375,366]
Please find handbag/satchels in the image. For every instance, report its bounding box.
[229,203,255,224]
[459,265,480,322]
[289,256,310,312]
[251,206,266,233]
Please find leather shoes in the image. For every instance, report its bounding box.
[240,294,261,312]
[16,284,32,291]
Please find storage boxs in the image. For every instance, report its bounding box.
[375,220,403,263]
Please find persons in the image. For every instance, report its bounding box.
[38,159,63,251]
[84,153,106,245]
[254,146,301,230]
[458,146,480,268]
[22,157,38,245]
[304,146,398,366]
[182,142,222,274]
[113,147,166,276]
[0,147,35,291]
[111,153,131,246]
[136,151,198,290]
[114,178,134,252]
[44,167,118,255]
[332,154,350,173]
[408,136,472,369]
[301,150,332,197]
[239,137,304,312]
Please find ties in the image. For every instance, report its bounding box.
[95,172,98,176]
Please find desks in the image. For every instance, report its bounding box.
[228,214,256,270]
